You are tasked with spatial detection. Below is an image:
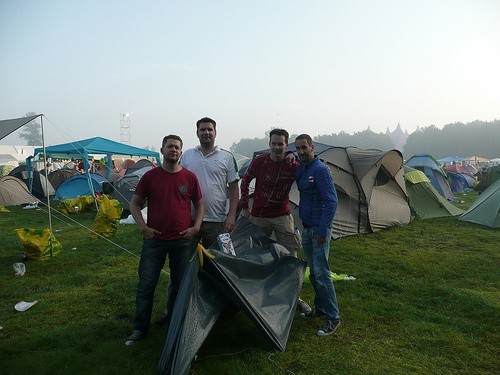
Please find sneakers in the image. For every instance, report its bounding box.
[300,309,323,318]
[317,320,340,336]
[124,332,143,345]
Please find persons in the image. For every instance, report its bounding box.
[180,117,240,248]
[240,129,332,256]
[284,133,341,335]
[127,135,204,344]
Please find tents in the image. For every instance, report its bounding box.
[230,141,500,239]
[160,214,309,375]
[0,113,161,257]
